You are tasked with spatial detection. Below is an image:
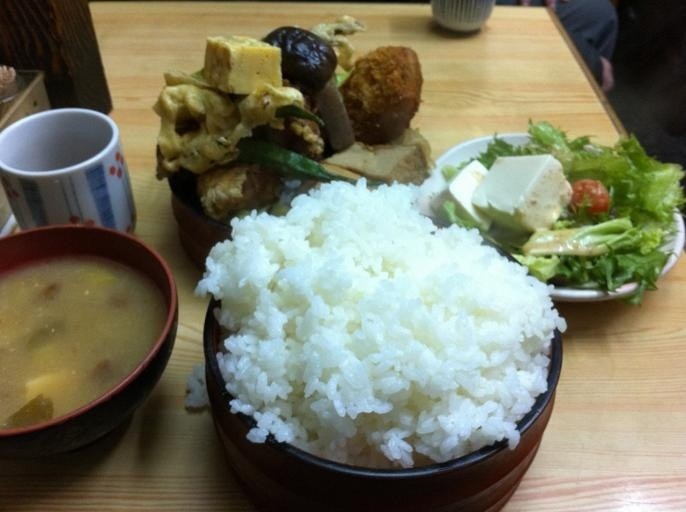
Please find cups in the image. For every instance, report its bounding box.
[0,107,137,234]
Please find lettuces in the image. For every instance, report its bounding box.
[439,120,686,307]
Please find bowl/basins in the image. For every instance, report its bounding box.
[204,239,562,511]
[0,224,177,455]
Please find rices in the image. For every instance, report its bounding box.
[191,178,568,475]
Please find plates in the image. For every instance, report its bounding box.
[430,133,685,300]
